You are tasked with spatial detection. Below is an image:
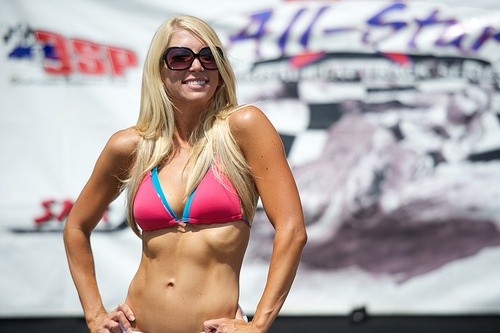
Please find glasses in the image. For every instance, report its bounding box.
[161,45,223,70]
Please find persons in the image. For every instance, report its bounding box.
[63,15,307,332]
